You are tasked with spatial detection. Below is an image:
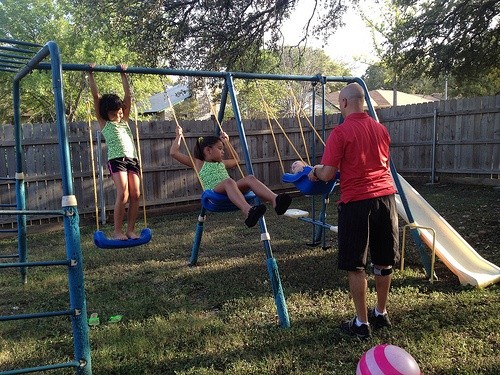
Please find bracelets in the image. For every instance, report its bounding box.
[313,168,317,177]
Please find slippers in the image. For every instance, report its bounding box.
[88,312,100,326]
[107,314,124,323]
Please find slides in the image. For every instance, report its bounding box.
[387,173,499,288]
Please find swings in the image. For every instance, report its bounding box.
[83,71,152,249]
[255,77,341,196]
[160,74,256,211]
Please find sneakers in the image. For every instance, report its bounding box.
[366,306,392,328]
[340,316,372,337]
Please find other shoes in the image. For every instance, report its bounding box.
[274,193,292,216]
[244,204,266,229]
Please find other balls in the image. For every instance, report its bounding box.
[356,345,421,375]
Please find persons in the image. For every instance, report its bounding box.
[290,160,340,185]
[313,85,403,339]
[169,126,292,227]
[88,64,141,240]
